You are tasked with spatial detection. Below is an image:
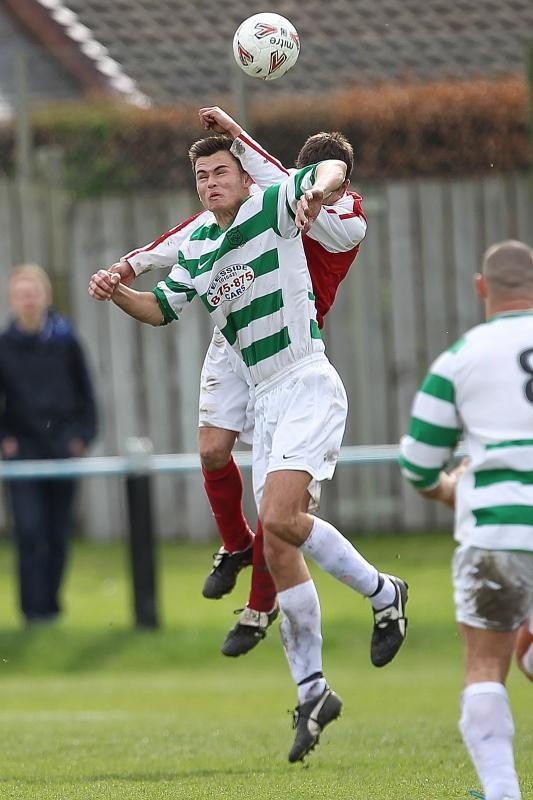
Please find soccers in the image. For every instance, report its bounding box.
[232,12,301,80]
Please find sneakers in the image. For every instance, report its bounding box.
[287,687,343,764]
[370,576,409,668]
[218,602,281,658]
[202,535,254,599]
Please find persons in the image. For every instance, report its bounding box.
[88,136,413,763]
[0,264,99,630]
[107,106,369,658]
[397,237,533,799]
